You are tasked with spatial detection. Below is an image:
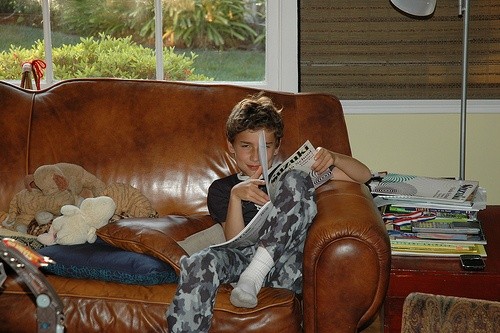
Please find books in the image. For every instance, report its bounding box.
[209,130,335,250]
[370,172,488,257]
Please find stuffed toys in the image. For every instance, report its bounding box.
[95,182,159,223]
[37,196,118,246]
[6,188,74,224]
[24,163,106,194]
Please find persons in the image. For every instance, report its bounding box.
[164,90,372,333]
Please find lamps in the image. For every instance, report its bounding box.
[388,0,470,180]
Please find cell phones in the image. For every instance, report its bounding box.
[460,255,486,271]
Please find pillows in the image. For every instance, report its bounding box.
[0,225,40,245]
[99,211,218,278]
[36,243,179,286]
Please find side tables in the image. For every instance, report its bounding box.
[385,205,500,333]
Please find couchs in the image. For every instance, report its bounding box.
[0,77,392,333]
